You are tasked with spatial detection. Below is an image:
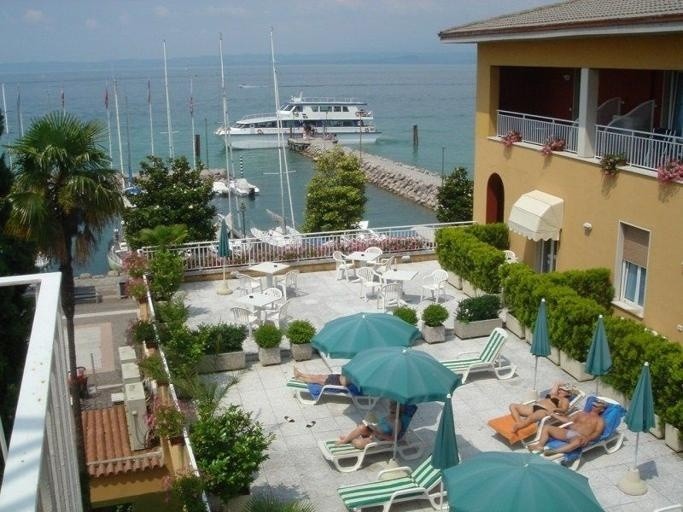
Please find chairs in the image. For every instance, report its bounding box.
[286,375,384,410]
[487,385,626,471]
[332,246,403,314]
[317,418,462,512]
[421,270,448,304]
[644,126,680,162]
[229,271,299,337]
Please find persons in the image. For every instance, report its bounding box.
[525,399,608,457]
[508,376,578,433]
[333,400,405,448]
[292,367,352,387]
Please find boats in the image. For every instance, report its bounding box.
[213,90,383,150]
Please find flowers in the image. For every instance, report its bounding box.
[538,135,565,158]
[655,158,683,185]
[498,130,522,148]
[600,151,626,176]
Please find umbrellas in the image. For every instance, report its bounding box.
[428,393,460,511]
[583,313,614,399]
[309,311,418,361]
[442,450,604,512]
[340,344,462,407]
[529,297,552,390]
[216,218,229,286]
[622,361,656,471]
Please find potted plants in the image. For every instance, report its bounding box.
[435,235,603,381]
[189,319,250,374]
[598,318,683,454]
[188,402,278,512]
[250,319,316,366]
[420,302,449,344]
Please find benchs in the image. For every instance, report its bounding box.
[74,286,98,303]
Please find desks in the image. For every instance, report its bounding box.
[382,270,420,302]
[247,261,291,289]
